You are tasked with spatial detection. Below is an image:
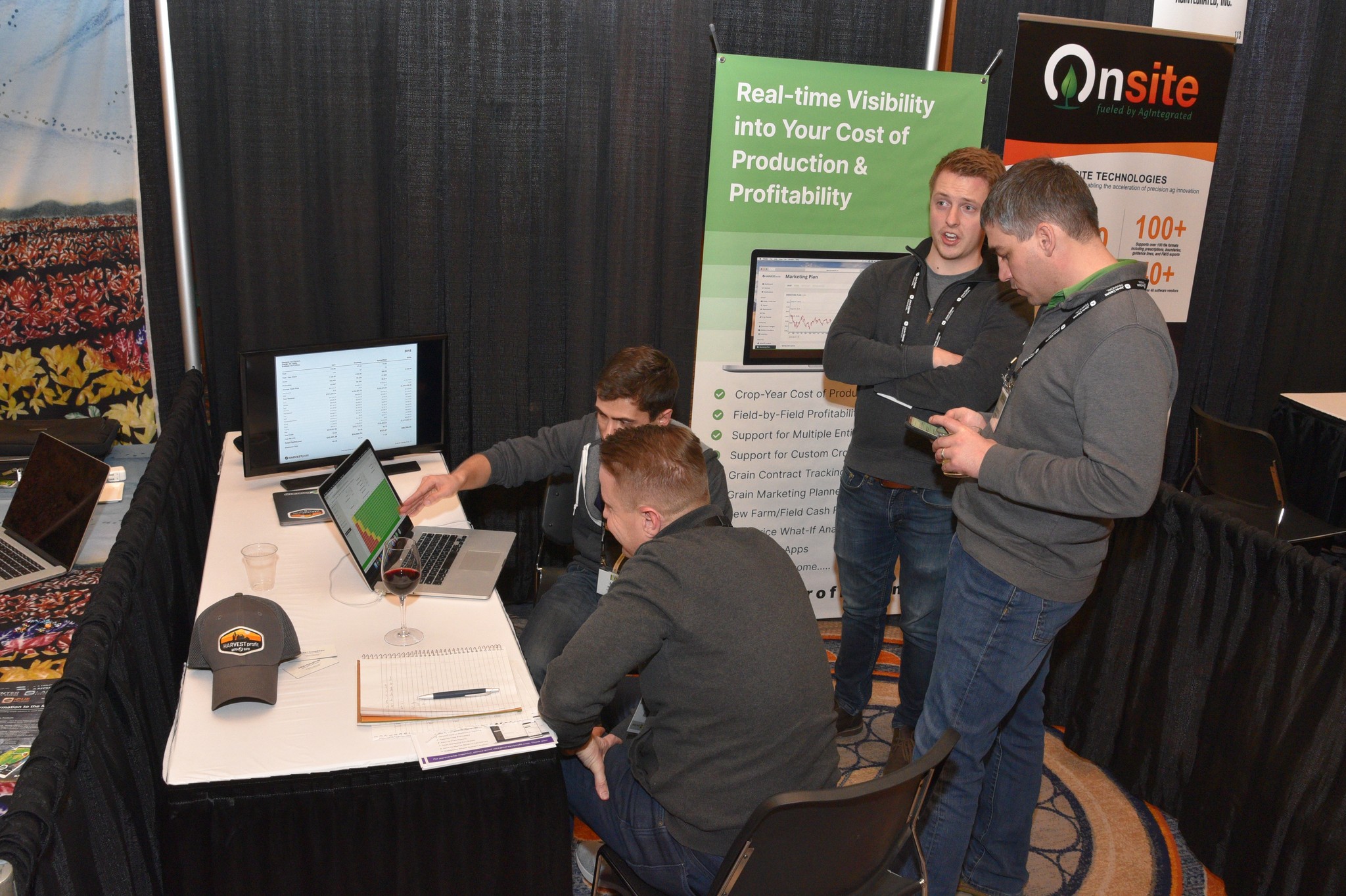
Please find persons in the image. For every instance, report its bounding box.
[892,156,1177,894]
[400,345,733,732]
[538,423,841,896]
[822,148,1033,776]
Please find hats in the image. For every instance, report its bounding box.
[188,592,302,711]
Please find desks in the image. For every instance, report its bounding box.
[1269,392,1346,529]
[162,432,577,896]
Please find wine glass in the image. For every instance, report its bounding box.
[381,537,425,647]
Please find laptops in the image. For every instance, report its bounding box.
[317,439,517,599]
[721,249,911,372]
[0,431,111,593]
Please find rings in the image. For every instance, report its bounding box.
[941,448,946,459]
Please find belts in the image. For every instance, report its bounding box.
[874,477,915,491]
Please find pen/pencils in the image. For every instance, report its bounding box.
[418,687,500,700]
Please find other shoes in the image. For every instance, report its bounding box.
[957,878,991,896]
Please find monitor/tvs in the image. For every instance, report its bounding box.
[237,332,445,491]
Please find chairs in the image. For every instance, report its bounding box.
[1180,405,1284,515]
[592,730,959,896]
[534,474,577,606]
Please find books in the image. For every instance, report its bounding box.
[356,644,522,725]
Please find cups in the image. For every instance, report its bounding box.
[242,542,279,591]
[937,426,981,478]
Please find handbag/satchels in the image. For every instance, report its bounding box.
[0,416,123,480]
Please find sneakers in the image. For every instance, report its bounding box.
[832,699,865,737]
[576,840,631,895]
[883,726,915,777]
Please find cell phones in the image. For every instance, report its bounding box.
[904,414,948,440]
